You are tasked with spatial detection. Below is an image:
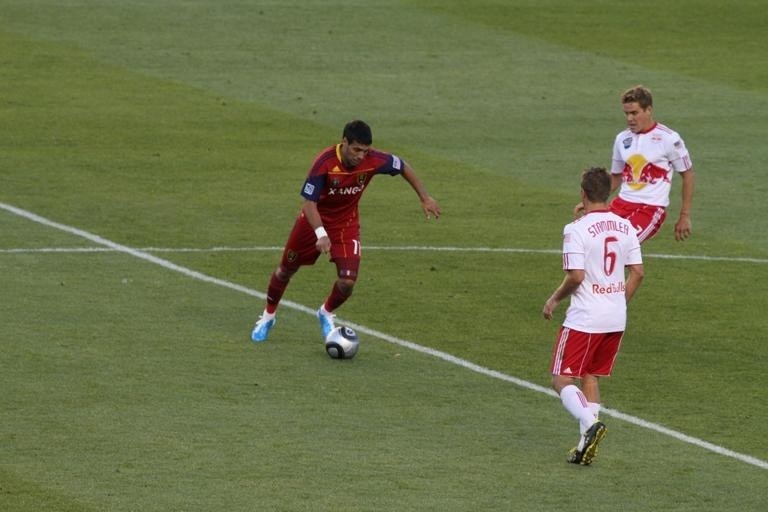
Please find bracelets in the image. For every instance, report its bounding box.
[314,226,328,238]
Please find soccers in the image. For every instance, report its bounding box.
[325,325,359,358]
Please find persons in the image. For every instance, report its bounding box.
[574,86,695,251]
[251,118,442,344]
[543,166,644,466]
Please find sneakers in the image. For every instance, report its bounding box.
[566,446,583,464]
[317,308,335,340]
[580,420,608,465]
[251,314,277,342]
